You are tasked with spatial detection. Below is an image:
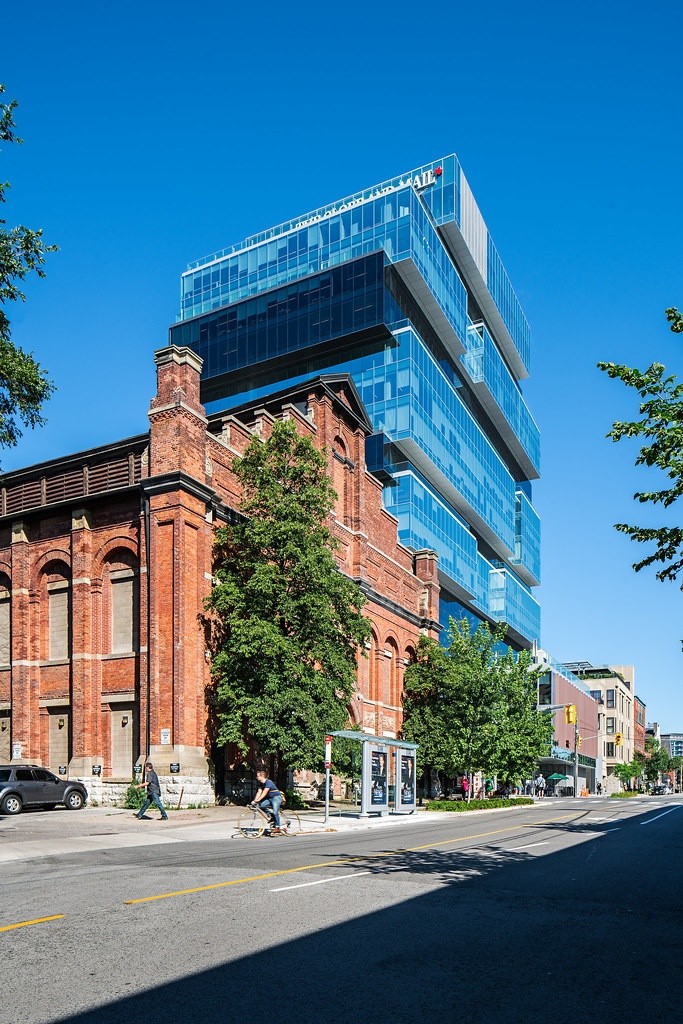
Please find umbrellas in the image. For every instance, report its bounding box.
[547,773,569,793]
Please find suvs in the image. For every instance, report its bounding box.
[1,762,90,815]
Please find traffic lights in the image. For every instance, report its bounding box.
[615,733,622,746]
[578,735,582,748]
[566,739,570,749]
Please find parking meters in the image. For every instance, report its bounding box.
[322,735,333,824]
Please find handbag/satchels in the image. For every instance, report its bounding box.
[536,785,539,791]
[279,791,286,805]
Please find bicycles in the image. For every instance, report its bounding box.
[237,801,301,839]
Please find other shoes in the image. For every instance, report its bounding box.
[157,818,168,821]
[271,828,280,833]
[133,813,141,819]
[267,818,274,823]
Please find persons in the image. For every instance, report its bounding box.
[484,776,494,797]
[407,758,414,783]
[536,773,546,799]
[132,763,168,820]
[377,753,386,777]
[250,770,283,833]
[597,781,601,795]
[459,774,468,801]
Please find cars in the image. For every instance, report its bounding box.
[650,786,666,795]
